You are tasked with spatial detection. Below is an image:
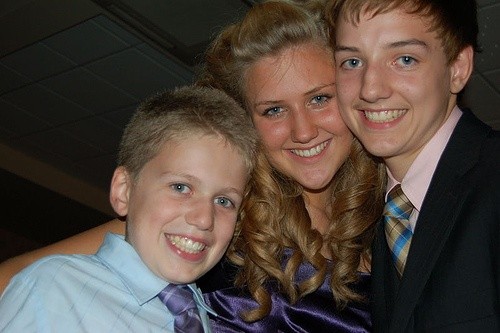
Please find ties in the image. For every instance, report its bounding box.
[383,185,414,277]
[157,283,205,333]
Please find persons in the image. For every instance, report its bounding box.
[0,82,258,333]
[0,0,390,333]
[324,0,500,333]
